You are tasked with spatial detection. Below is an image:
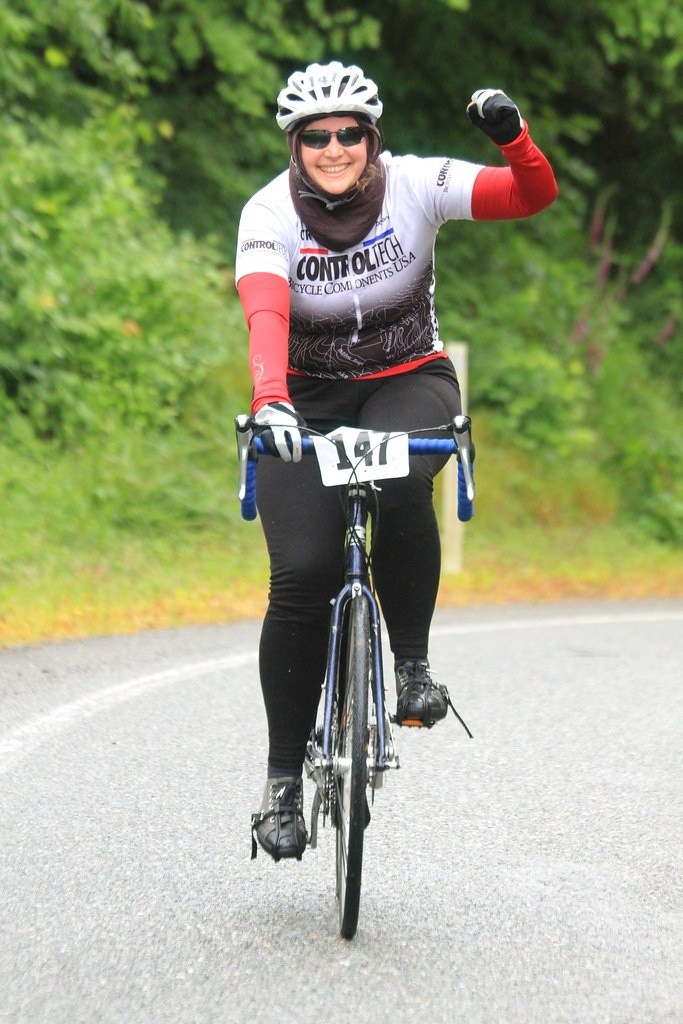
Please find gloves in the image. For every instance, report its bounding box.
[254,401,306,463]
[466,88,524,144]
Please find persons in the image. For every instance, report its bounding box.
[233,60,558,862]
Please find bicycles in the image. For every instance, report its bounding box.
[236,416,474,943]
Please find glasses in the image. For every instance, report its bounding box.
[299,125,369,150]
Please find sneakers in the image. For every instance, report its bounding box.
[394,659,447,720]
[257,775,306,857]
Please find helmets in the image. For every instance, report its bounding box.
[276,60,384,132]
